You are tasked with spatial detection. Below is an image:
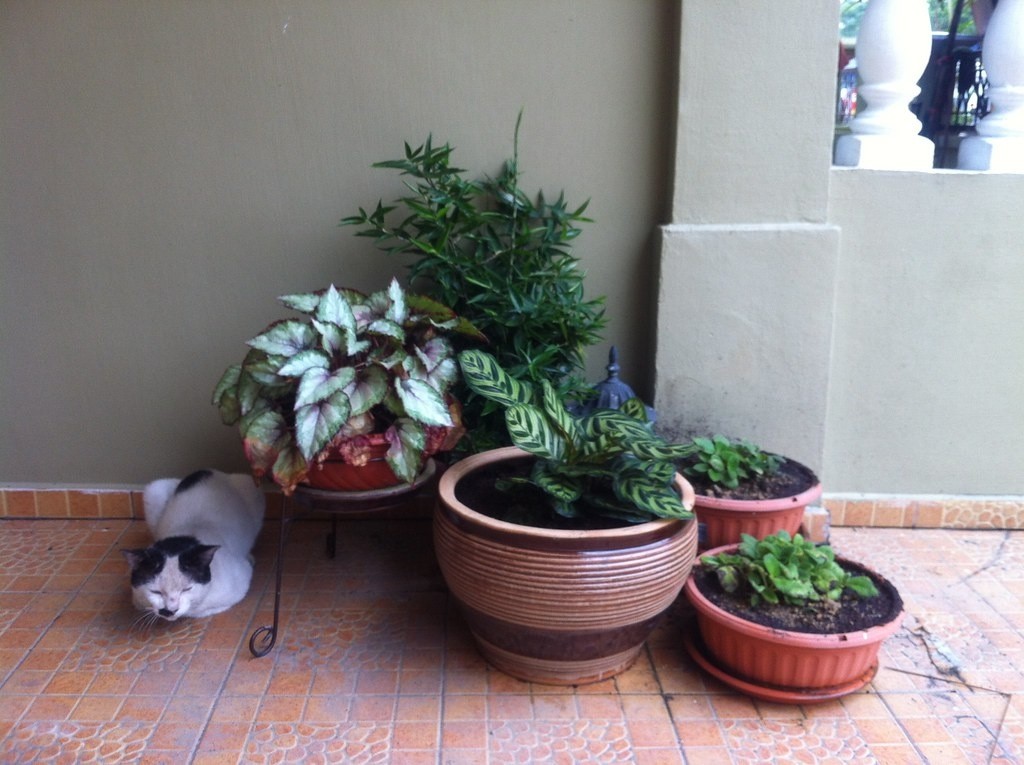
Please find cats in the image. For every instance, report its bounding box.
[116,467,265,636]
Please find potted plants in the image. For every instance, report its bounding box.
[674,432,820,546]
[431,343,697,686]
[215,288,489,496]
[685,540,904,704]
